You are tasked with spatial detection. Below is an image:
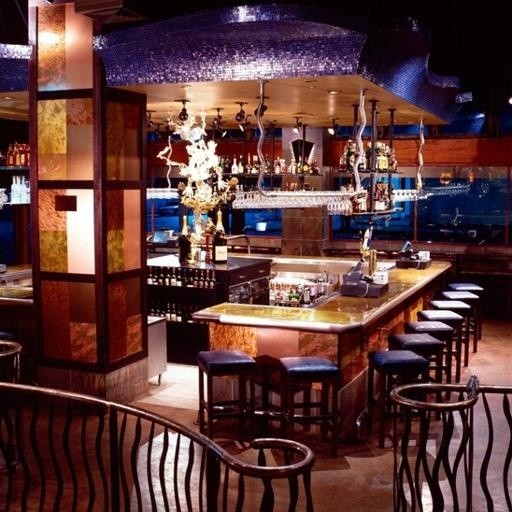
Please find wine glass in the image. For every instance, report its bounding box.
[391,188,420,203]
[232,188,354,216]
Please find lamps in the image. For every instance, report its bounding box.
[292,116,302,133]
[327,119,339,135]
[174,96,271,137]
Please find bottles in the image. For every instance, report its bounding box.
[339,138,392,214]
[269,279,325,304]
[6,142,32,205]
[147,266,217,325]
[211,210,228,265]
[217,153,322,176]
[178,215,192,266]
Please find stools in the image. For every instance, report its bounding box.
[198,348,338,459]
[368,283,484,449]
